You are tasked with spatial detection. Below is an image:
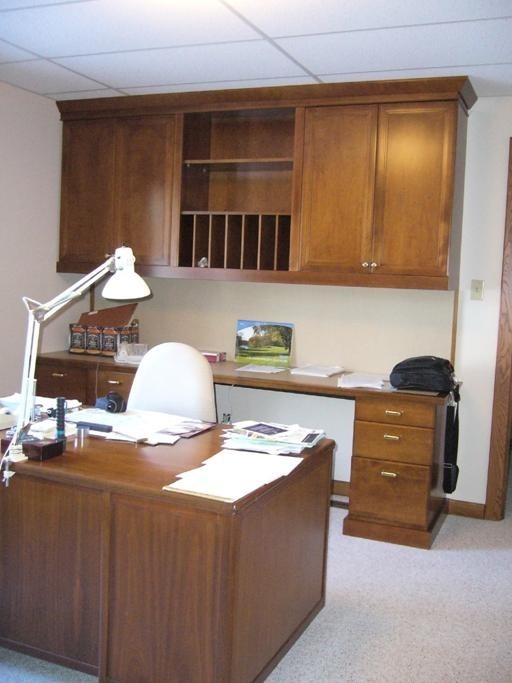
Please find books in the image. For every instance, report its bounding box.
[0,389,215,448]
[288,361,390,389]
[219,417,327,457]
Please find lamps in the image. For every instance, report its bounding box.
[0,245,151,463]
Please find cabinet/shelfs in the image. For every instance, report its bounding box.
[55,93,181,282]
[182,86,295,281]
[298,74,476,291]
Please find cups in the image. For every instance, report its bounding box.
[75,426,90,448]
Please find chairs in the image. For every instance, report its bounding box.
[127,340,219,422]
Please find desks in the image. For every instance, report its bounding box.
[27,348,463,549]
[0,394,336,682]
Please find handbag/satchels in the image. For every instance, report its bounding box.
[389,356,458,390]
